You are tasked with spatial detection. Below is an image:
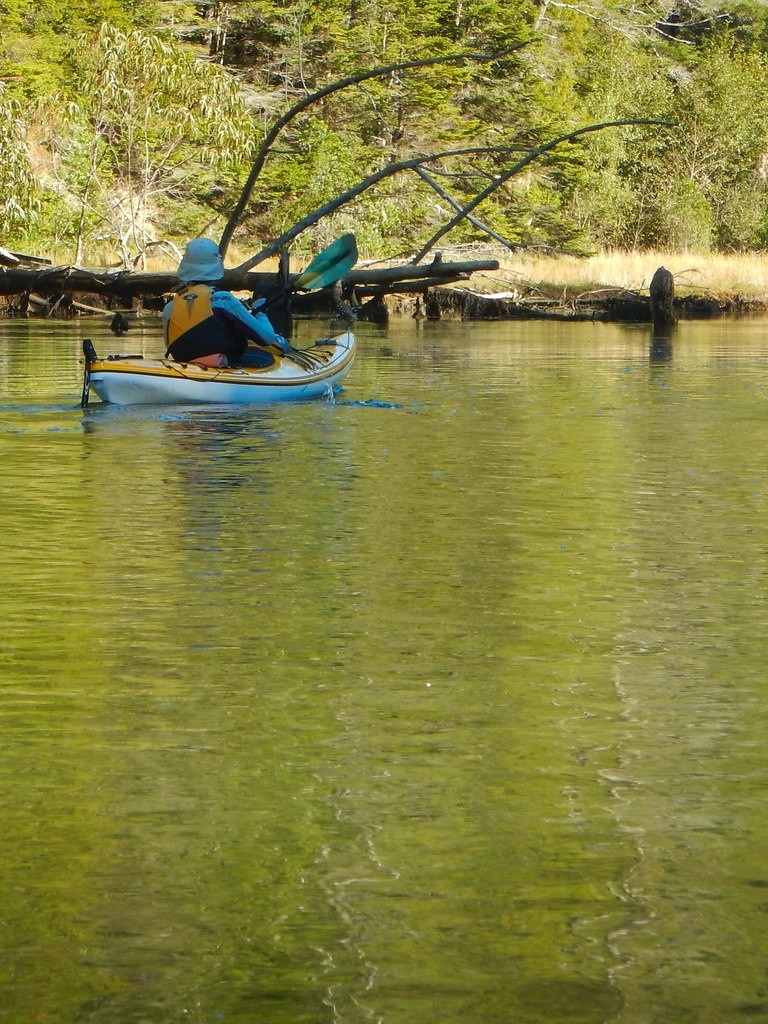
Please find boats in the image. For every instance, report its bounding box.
[79,328,358,404]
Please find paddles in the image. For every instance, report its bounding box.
[248,233,359,317]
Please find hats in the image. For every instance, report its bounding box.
[177,237,224,281]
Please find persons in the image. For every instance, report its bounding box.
[162,238,286,369]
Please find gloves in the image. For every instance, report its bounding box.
[251,298,268,313]
